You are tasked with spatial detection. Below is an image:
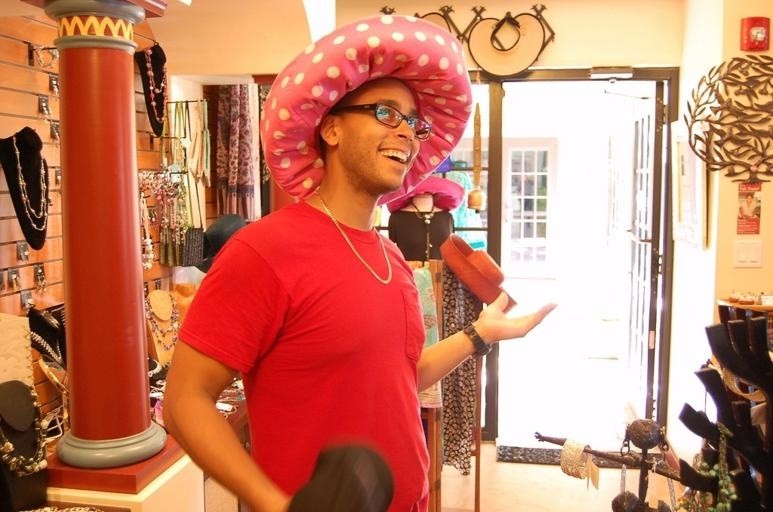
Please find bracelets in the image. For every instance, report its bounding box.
[463,322,494,359]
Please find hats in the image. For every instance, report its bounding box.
[256,12,474,208]
[438,232,518,314]
[386,176,466,212]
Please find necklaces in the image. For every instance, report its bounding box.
[7,131,50,232]
[142,48,171,123]
[678,421,743,512]
[410,199,438,261]
[147,359,163,380]
[0,385,51,478]
[145,293,181,350]
[312,188,394,286]
[137,165,189,272]
[27,310,64,365]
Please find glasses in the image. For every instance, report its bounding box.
[328,101,433,142]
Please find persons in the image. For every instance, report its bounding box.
[386,191,457,262]
[737,192,762,220]
[161,79,562,510]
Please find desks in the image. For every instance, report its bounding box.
[215,386,250,430]
[419,376,441,512]
